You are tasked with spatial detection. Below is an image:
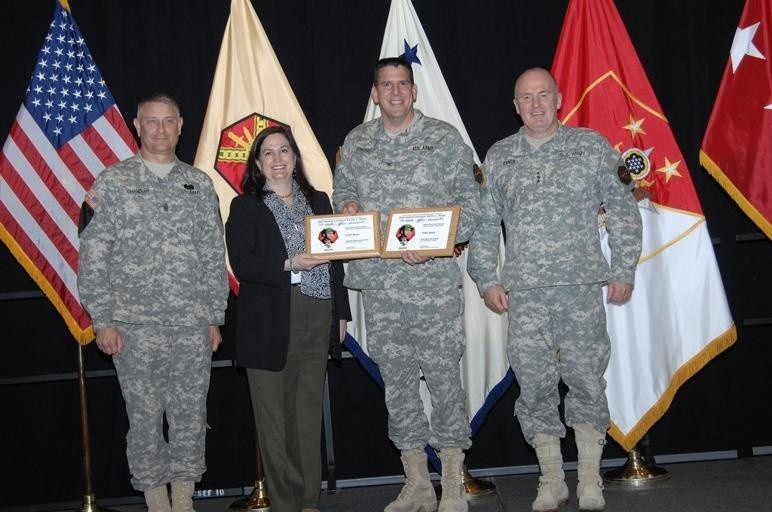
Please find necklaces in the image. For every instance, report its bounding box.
[265,182,293,198]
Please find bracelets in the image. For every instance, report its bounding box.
[289,255,299,274]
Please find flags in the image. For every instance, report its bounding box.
[463,66,644,512]
[193,0,334,298]
[1,0,138,347]
[344,0,515,478]
[699,0,772,237]
[550,1,737,456]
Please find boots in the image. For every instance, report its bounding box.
[437,445,470,512]
[571,426,607,510]
[379,447,440,512]
[168,478,197,512]
[143,483,173,512]
[529,430,574,511]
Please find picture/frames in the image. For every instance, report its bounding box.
[302,206,461,261]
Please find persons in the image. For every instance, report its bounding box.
[224,122,354,511]
[75,92,232,511]
[330,55,485,512]
[397,223,414,243]
[320,227,336,245]
